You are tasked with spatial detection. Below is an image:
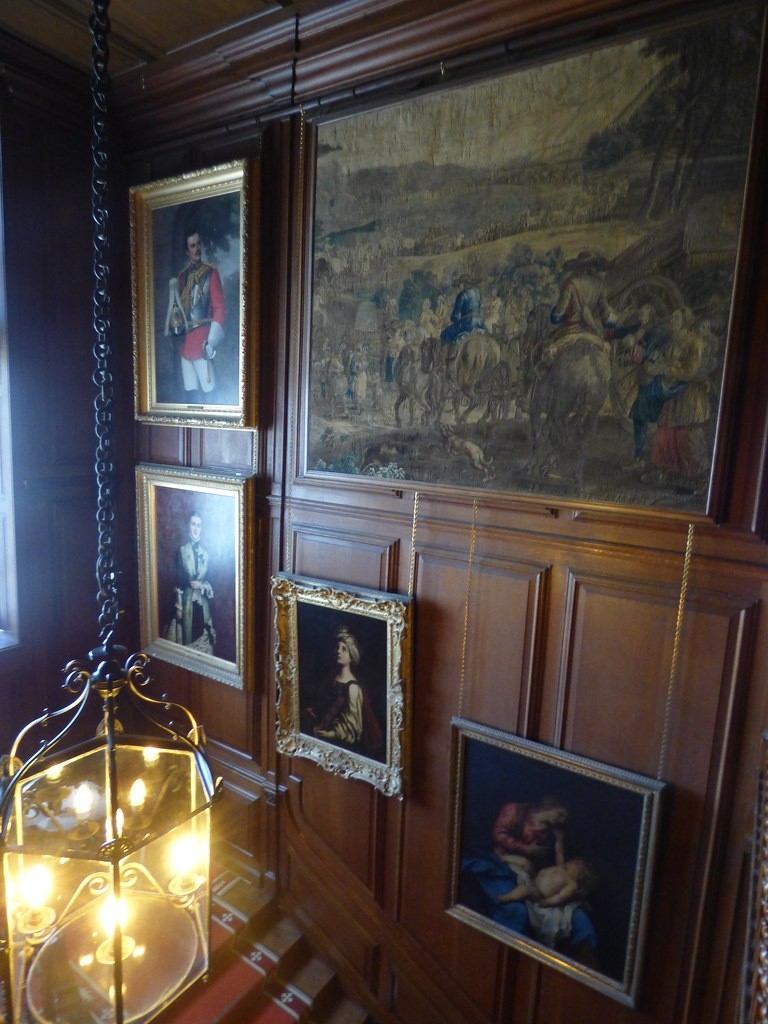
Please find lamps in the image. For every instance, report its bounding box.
[0,0,223,1024]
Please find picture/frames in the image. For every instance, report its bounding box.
[133,461,259,696]
[125,156,260,432]
[440,715,667,1009]
[269,571,411,805]
[297,0,768,530]
